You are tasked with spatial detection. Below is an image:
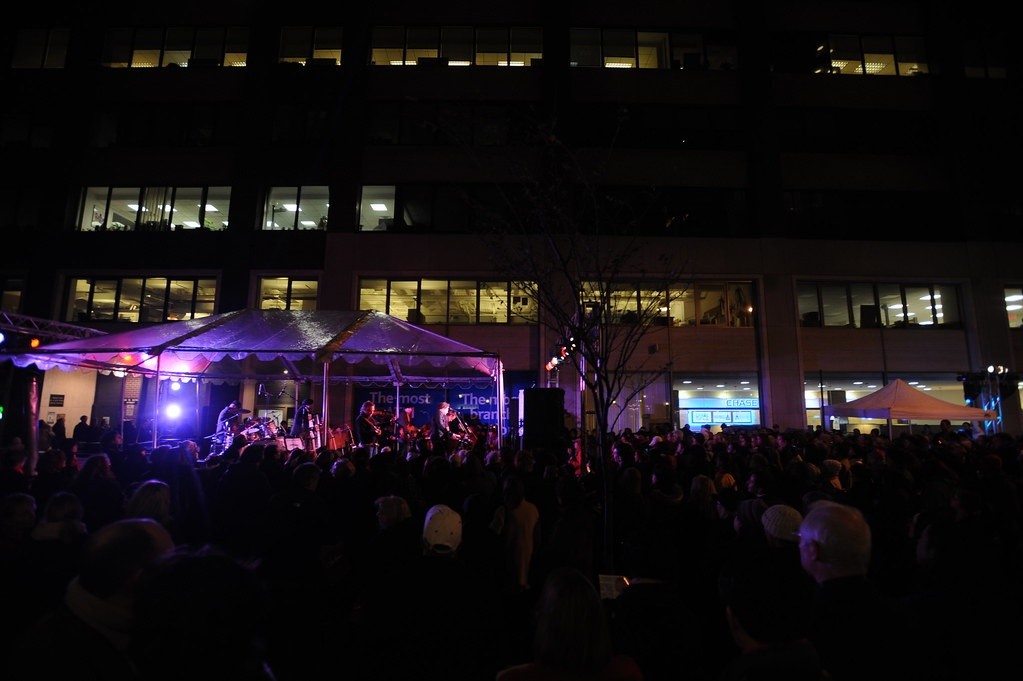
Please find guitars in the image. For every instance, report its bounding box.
[365,418,382,438]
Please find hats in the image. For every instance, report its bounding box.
[760,504,803,542]
[422,505,462,555]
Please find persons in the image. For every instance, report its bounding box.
[0,397,1023,681]
[14,375,39,475]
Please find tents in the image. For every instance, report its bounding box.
[0,310,503,452]
[822,378,998,437]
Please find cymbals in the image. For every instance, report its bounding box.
[230,408,251,414]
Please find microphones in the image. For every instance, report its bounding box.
[257,384,262,395]
[275,385,287,399]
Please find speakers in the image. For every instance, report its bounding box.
[518,387,565,457]
